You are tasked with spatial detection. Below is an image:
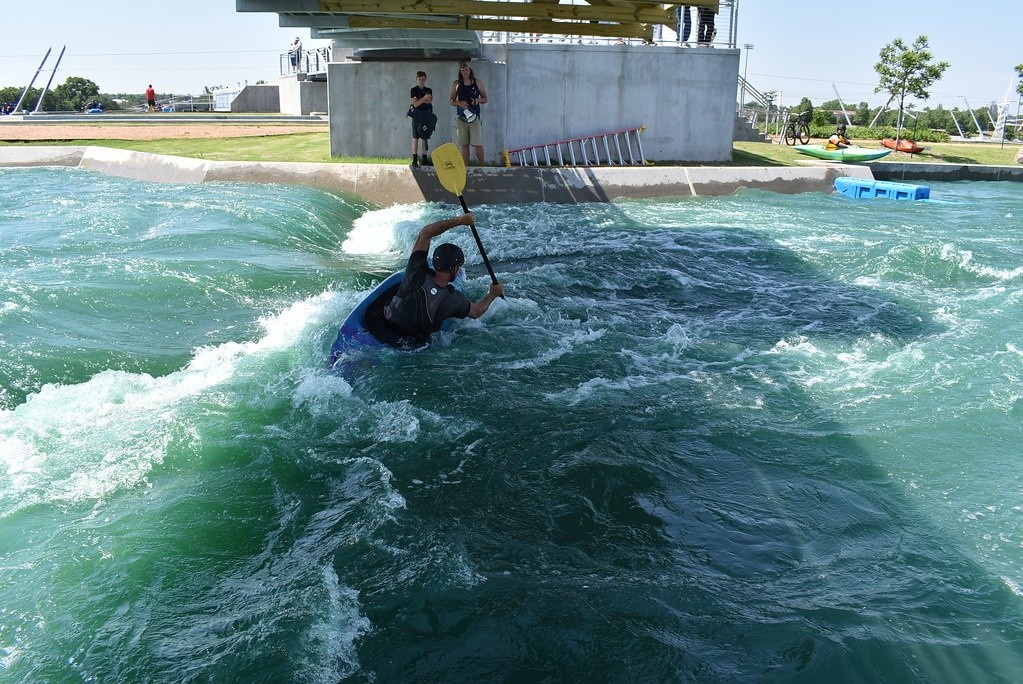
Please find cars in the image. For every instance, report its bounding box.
[0,102,17,114]
[136,96,200,112]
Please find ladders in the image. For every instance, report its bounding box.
[503,126,655,168]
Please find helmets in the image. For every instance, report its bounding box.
[433,243,464,271]
[838,124,847,130]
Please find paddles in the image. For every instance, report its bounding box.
[826,143,877,151]
[431,141,505,301]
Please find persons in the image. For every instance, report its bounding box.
[410,71,435,166]
[826,124,851,151]
[676,5,691,47]
[450,62,487,167]
[697,0,719,47]
[288,37,302,73]
[384,213,506,340]
[145,84,158,113]
[79,100,106,114]
[4,104,12,115]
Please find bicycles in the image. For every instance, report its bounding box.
[784,111,810,145]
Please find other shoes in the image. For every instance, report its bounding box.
[697,44,713,47]
[421,160,433,166]
[677,42,690,47]
[412,162,418,167]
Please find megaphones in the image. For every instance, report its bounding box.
[458,106,477,123]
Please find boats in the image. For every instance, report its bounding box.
[327,254,466,382]
[880,136,924,153]
[790,144,894,162]
[85,108,103,114]
